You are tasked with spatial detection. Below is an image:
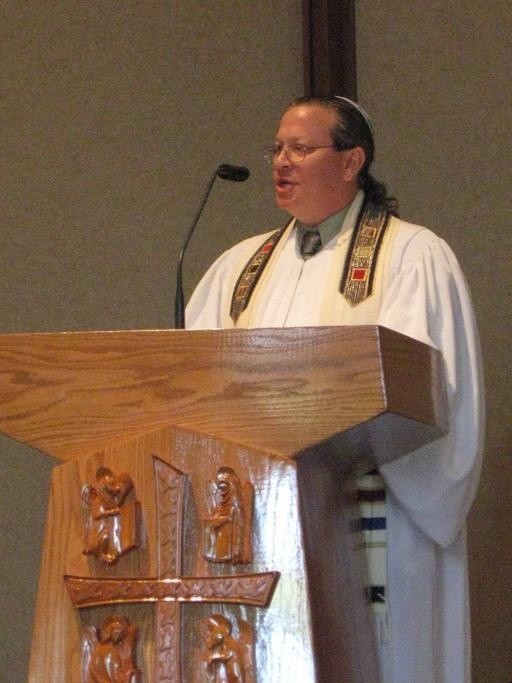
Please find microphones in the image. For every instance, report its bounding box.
[174,163,251,329]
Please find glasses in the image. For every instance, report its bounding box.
[261,144,336,164]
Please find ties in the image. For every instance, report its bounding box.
[300,231,322,262]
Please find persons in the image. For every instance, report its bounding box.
[83,476,120,563]
[205,615,245,683]
[184,94,487,683]
[87,615,138,683]
[202,466,244,562]
